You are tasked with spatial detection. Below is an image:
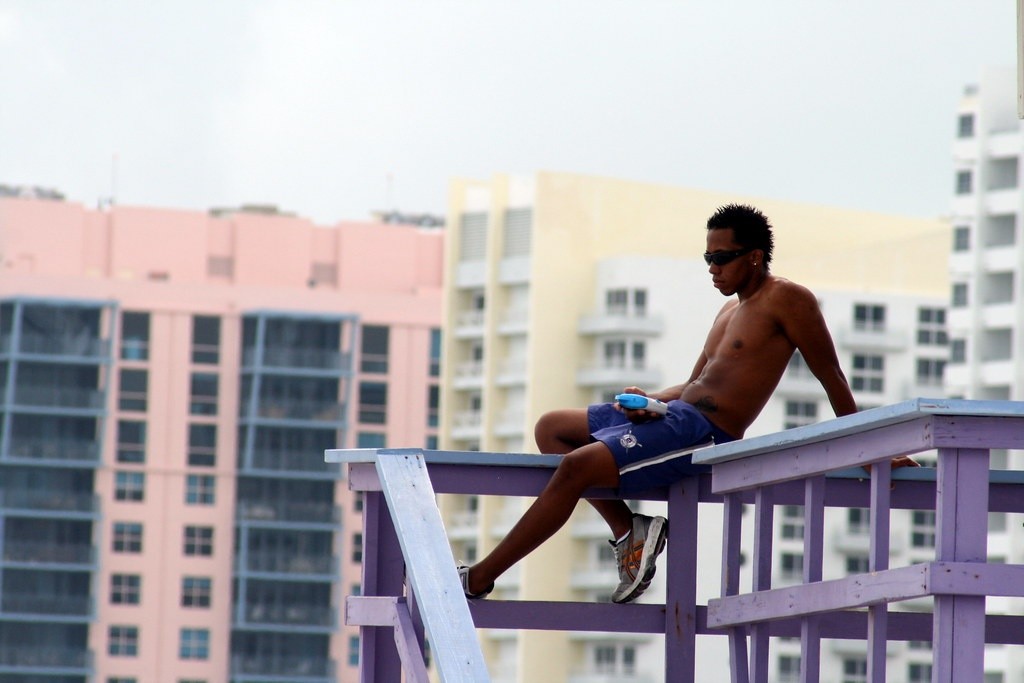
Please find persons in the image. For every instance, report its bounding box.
[404,204,921,603]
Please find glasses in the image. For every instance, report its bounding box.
[704,246,764,266]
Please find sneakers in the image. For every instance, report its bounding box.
[608,513,667,604]
[403,559,494,599]
